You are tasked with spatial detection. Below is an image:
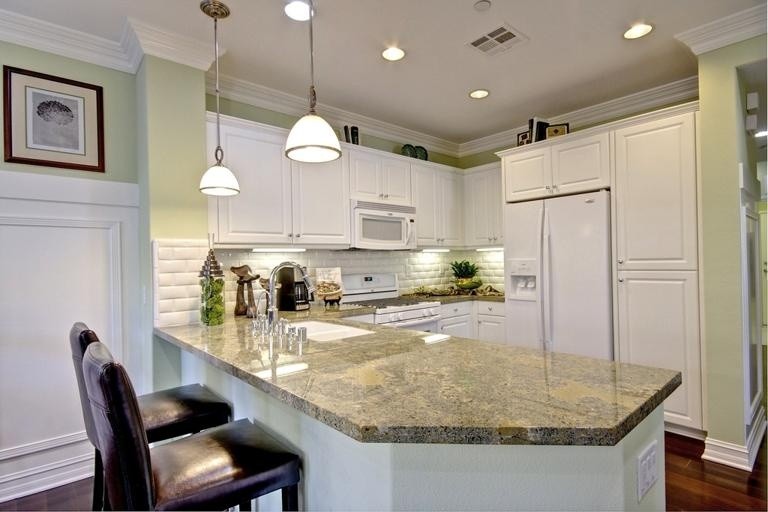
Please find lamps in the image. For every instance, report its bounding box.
[198,0,241,197]
[284,1,342,164]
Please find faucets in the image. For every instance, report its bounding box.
[268,262,315,324]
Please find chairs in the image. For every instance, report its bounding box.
[69,320,231,512]
[82,341,303,512]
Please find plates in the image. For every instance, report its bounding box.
[400,143,417,158]
[412,146,428,160]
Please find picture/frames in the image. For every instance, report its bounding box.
[4,64,105,173]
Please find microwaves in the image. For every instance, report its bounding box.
[353,208,418,251]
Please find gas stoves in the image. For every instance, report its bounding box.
[341,295,442,325]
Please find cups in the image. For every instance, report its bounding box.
[308,300,326,314]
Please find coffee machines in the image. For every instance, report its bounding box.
[276,265,312,312]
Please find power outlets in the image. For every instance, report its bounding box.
[637,440,659,502]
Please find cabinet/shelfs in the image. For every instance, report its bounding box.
[493,123,610,203]
[610,99,708,444]
[465,160,504,248]
[476,298,506,345]
[208,114,349,252]
[437,298,475,340]
[153,307,682,512]
[411,157,464,250]
[348,143,412,204]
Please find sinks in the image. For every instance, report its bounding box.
[278,320,343,335]
[310,328,376,342]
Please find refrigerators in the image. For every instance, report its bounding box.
[504,190,614,363]
